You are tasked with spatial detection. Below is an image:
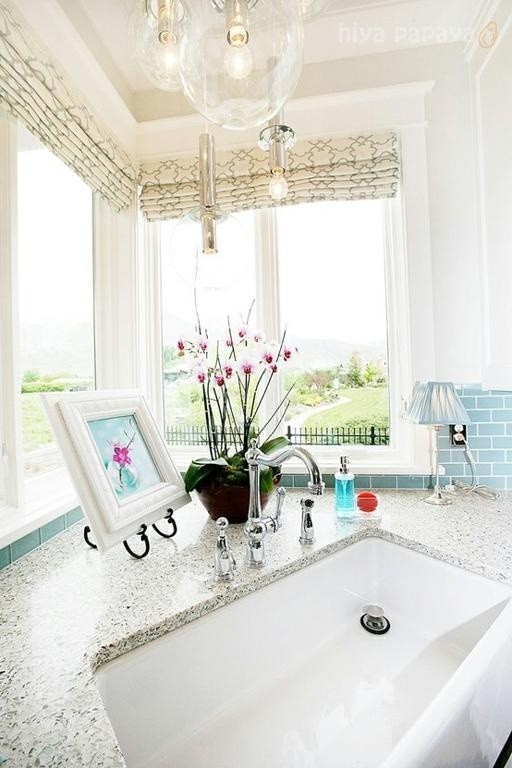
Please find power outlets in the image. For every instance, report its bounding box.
[449,423,468,447]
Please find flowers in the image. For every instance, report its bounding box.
[174,248,302,494]
[111,419,136,488]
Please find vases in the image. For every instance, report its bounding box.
[107,439,138,493]
[194,475,281,524]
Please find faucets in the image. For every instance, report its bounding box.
[241,435,325,565]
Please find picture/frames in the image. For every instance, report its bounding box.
[39,385,191,554]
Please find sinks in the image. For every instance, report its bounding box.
[88,529,512,768]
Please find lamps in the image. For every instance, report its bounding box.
[127,1,331,257]
[401,379,473,505]
